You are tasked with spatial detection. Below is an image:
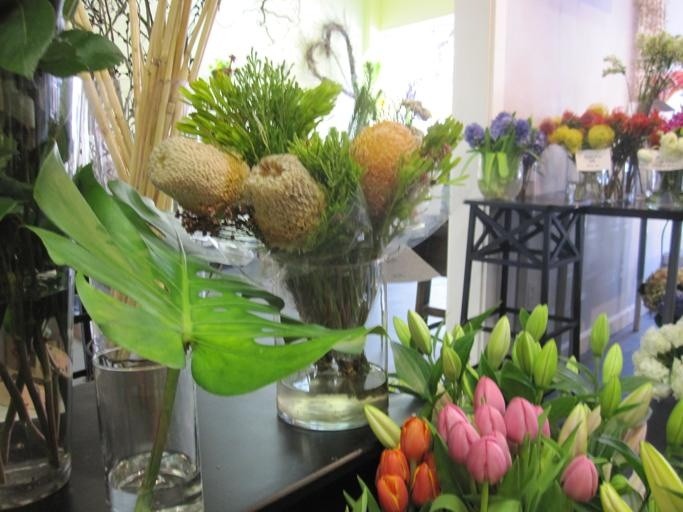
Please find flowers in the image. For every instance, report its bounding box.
[461,102,682,153]
[342,300,683,511]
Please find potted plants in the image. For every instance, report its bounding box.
[0,0,391,512]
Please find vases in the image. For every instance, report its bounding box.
[471,146,681,211]
[253,245,400,432]
